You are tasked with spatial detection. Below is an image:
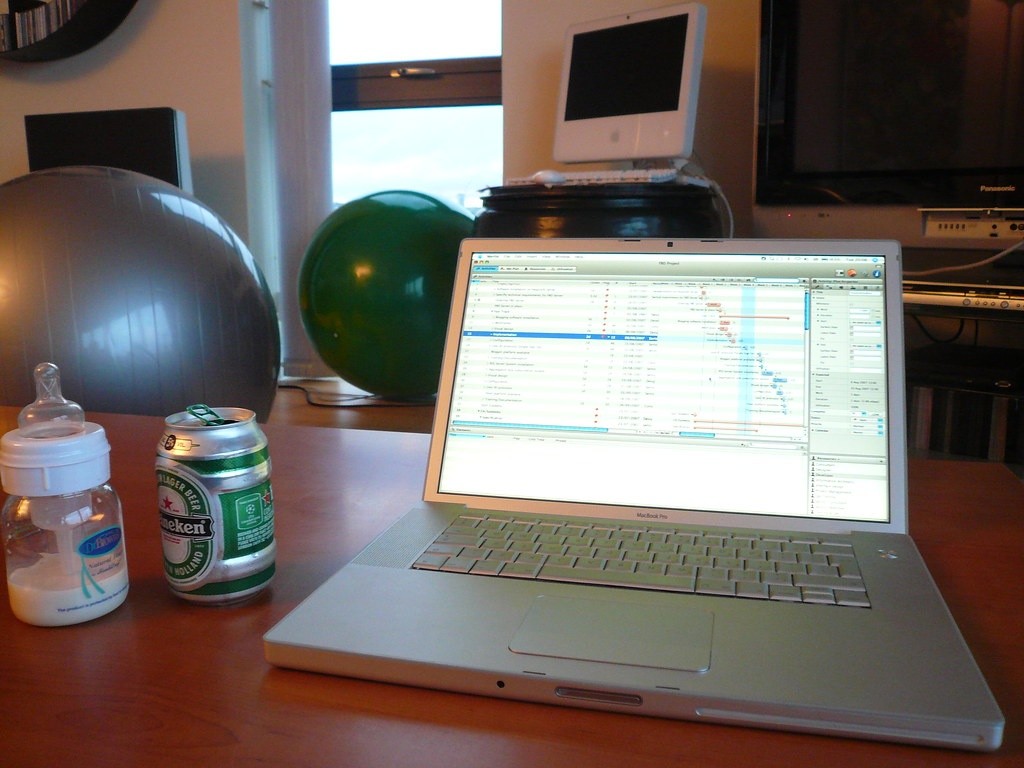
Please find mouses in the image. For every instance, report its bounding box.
[532,169,568,185]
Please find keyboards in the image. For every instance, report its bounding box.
[504,168,677,183]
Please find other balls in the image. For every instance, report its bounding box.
[1,165,281,424]
[299,190,478,399]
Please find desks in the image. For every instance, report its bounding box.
[0,406,1024,767]
[905,342,1024,464]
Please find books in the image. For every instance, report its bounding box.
[0,14,11,52]
[16,0,81,53]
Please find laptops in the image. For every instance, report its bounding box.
[264,237,1001,748]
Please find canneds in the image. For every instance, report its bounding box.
[153,404,276,605]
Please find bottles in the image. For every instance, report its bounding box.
[0,362,130,627]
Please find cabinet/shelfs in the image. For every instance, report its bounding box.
[472,185,723,239]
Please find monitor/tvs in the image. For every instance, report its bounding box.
[553,2,707,168]
[755,0,1024,254]
